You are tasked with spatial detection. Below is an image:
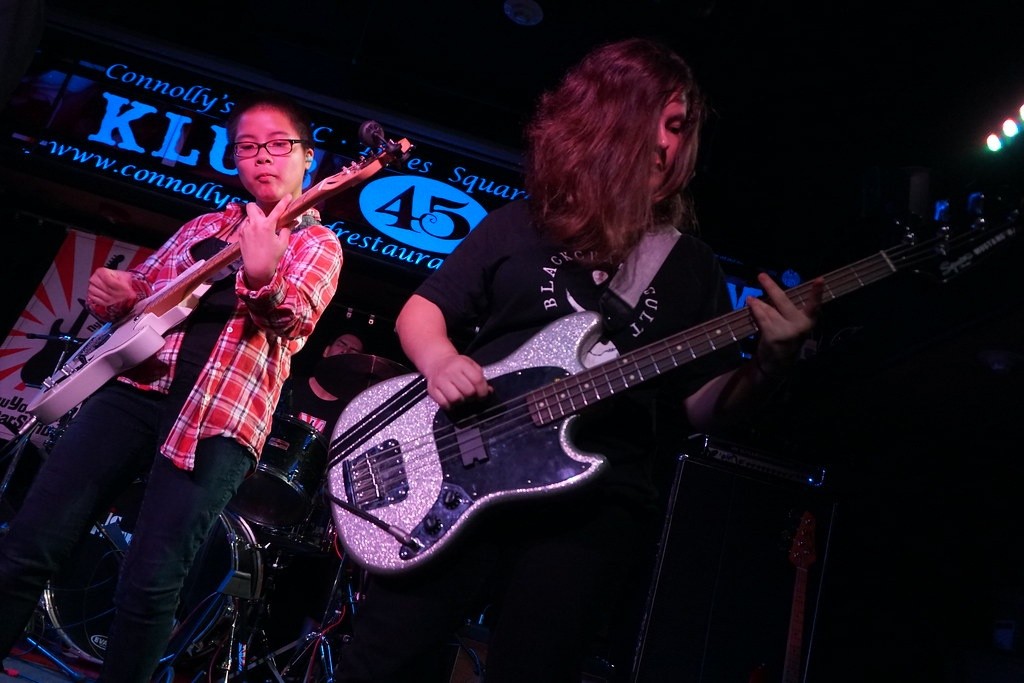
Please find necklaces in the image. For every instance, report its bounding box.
[213,215,247,249]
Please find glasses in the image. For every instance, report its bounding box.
[232,139,306,159]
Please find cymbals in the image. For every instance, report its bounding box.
[314,352,416,403]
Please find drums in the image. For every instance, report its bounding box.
[252,502,336,557]
[224,411,329,529]
[42,478,264,666]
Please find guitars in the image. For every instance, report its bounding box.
[25,117,416,428]
[325,184,1024,578]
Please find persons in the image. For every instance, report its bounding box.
[328,35,824,683]
[0,92,342,683]
[277,328,367,442]
